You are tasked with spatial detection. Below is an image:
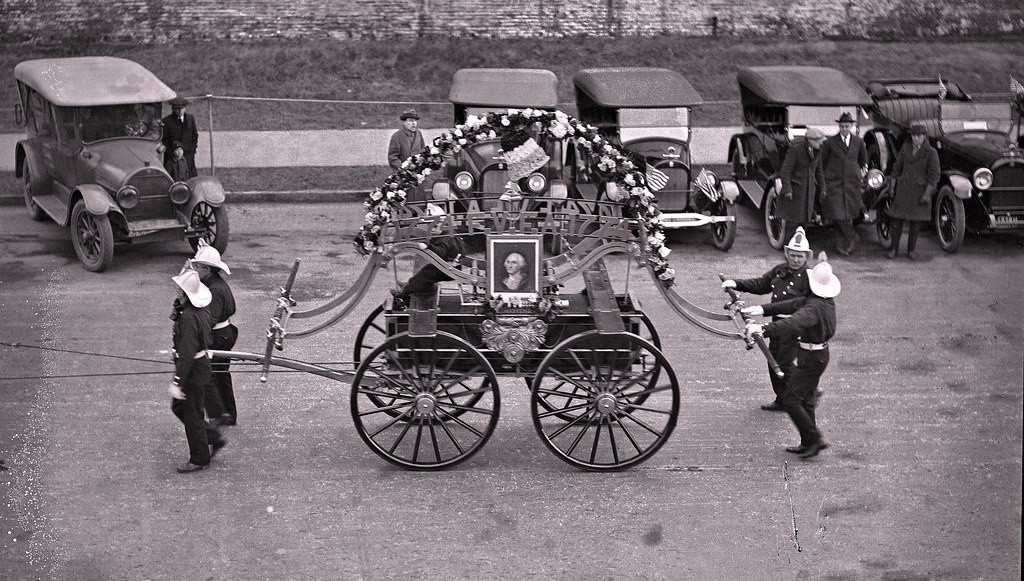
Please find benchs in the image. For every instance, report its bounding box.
[879,99,962,125]
[908,118,965,140]
[743,107,787,126]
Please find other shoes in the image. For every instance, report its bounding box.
[797,438,830,459]
[906,250,919,259]
[886,248,899,259]
[176,458,210,473]
[846,234,860,251]
[207,435,232,457]
[208,413,238,428]
[831,246,851,258]
[787,444,809,453]
[760,400,788,412]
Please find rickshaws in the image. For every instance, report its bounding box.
[199,109,788,472]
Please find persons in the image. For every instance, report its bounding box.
[822,112,868,256]
[168,244,238,473]
[162,97,198,182]
[389,203,464,301]
[388,109,425,202]
[884,125,940,260]
[741,262,841,458]
[777,127,828,243]
[723,231,813,412]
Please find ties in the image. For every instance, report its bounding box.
[844,136,848,145]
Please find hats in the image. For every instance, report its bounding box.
[835,113,856,124]
[398,108,421,120]
[782,225,814,260]
[168,95,191,110]
[906,125,929,135]
[802,249,841,299]
[804,127,824,139]
[191,237,232,275]
[170,141,185,151]
[171,260,215,308]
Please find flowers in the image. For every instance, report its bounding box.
[350,106,677,289]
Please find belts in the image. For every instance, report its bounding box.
[169,349,207,361]
[775,314,792,319]
[210,317,235,330]
[796,340,828,351]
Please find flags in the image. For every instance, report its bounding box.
[646,163,668,192]
[693,167,721,202]
[1010,78,1024,94]
[938,78,947,99]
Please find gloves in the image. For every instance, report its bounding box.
[890,177,897,202]
[920,183,939,206]
[743,322,764,345]
[742,302,765,318]
[722,279,737,294]
[167,383,186,400]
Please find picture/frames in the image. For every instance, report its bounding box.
[486,233,542,300]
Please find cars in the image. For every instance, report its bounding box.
[726,64,903,252]
[574,66,737,254]
[443,67,564,246]
[15,57,230,273]
[870,73,1024,254]
